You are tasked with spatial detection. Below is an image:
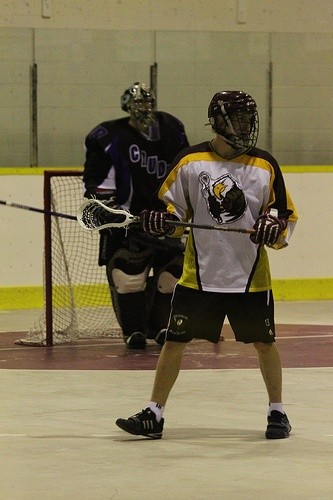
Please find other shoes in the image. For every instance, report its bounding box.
[125,331,148,350]
[154,328,167,345]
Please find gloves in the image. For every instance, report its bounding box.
[139,209,170,242]
[250,213,284,247]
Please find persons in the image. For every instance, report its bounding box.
[116,91,299,439]
[83,80,190,348]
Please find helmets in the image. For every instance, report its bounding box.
[204,91,260,155]
[121,81,156,129]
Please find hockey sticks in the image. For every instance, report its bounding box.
[76,199,255,233]
[0,199,78,220]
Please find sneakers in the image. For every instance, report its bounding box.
[115,407,165,439]
[264,410,292,439]
